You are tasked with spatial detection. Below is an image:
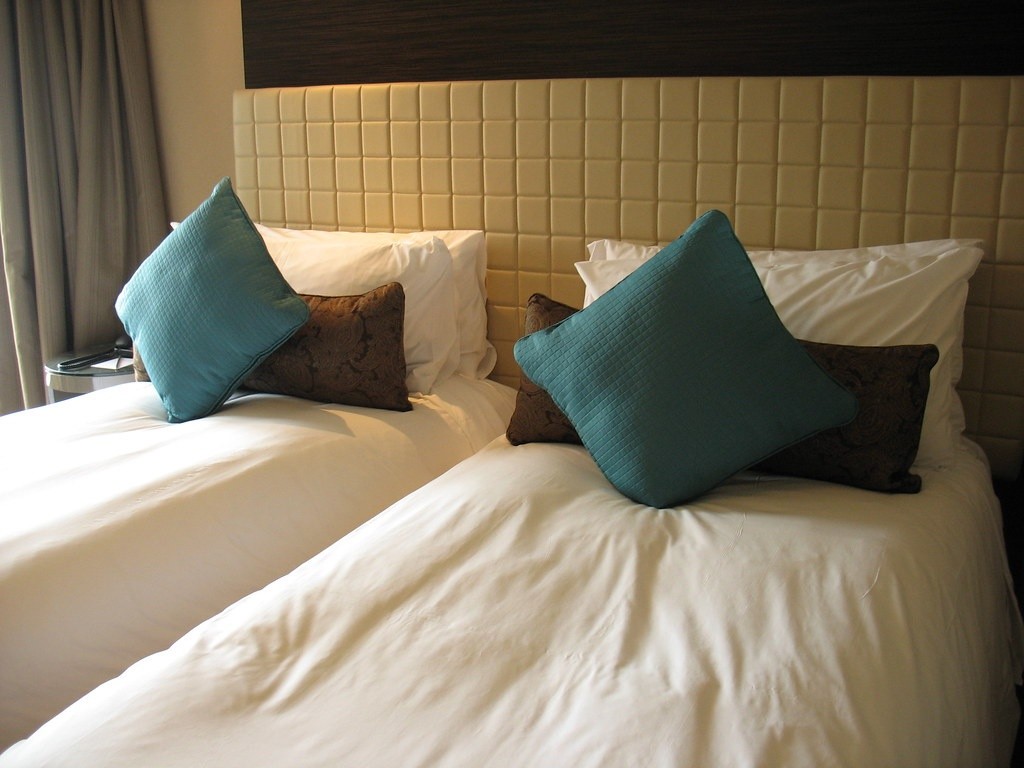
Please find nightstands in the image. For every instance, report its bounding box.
[45,345,135,405]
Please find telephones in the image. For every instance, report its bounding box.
[114,335,135,359]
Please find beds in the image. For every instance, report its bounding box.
[0,380,522,754]
[0,435,1024,768]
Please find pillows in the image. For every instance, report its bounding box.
[237,282,413,413]
[514,208,859,508]
[169,221,462,396]
[131,341,152,382]
[586,238,986,453]
[114,177,310,423]
[270,227,497,381]
[506,293,584,446]
[741,339,941,495]
[572,248,987,466]
